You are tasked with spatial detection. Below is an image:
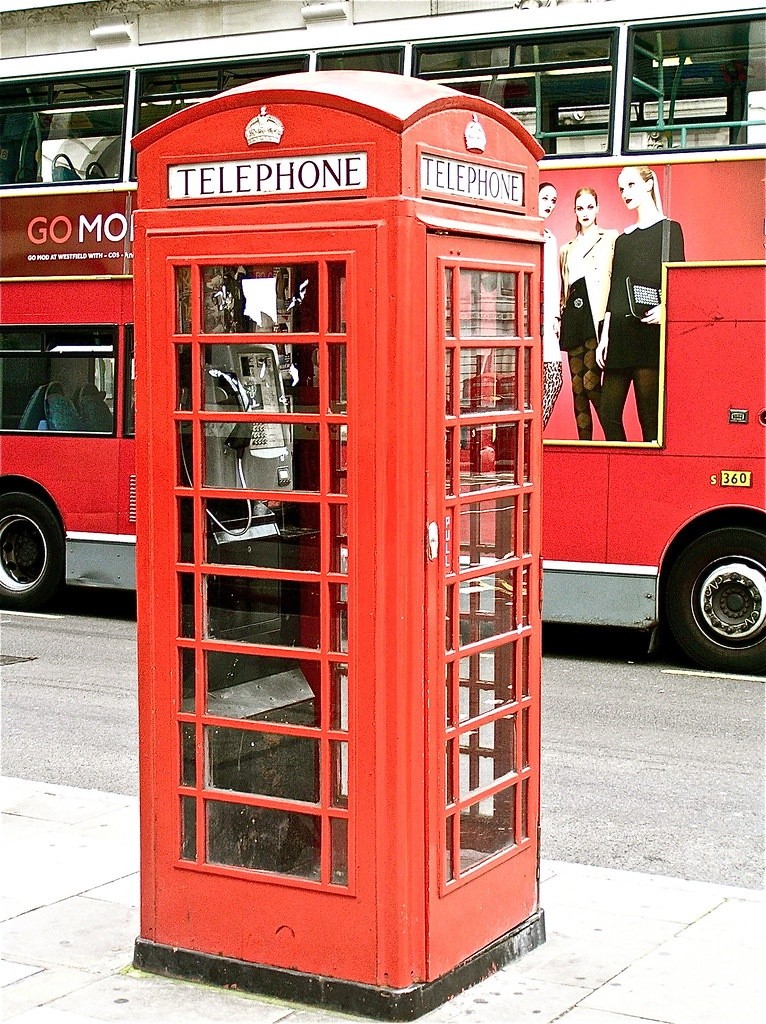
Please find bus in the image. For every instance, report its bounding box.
[1,5,763,674]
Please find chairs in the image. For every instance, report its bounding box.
[16,382,51,430]
[51,155,82,185]
[14,166,35,183]
[77,383,113,433]
[82,158,109,181]
[42,380,86,432]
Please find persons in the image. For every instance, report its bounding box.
[516,164,688,443]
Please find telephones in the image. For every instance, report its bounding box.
[176,342,293,537]
